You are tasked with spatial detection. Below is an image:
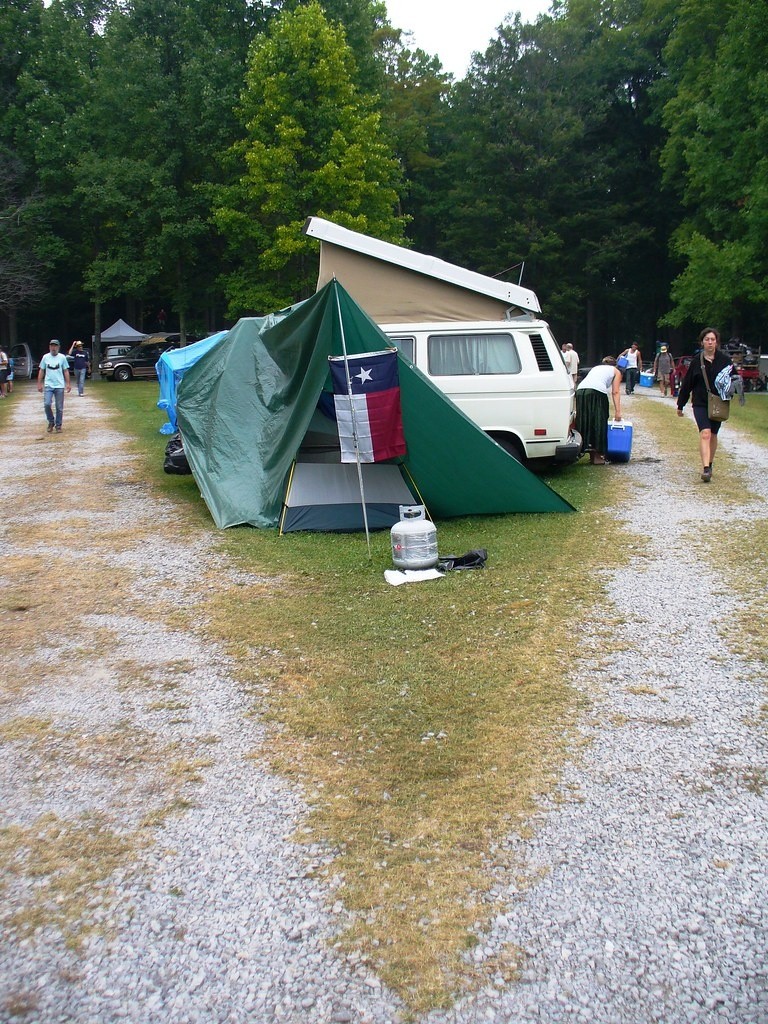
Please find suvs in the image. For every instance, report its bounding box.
[98,345,133,362]
[98,341,196,382]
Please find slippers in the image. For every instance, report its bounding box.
[590,456,611,465]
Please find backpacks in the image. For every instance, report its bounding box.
[1,353,11,375]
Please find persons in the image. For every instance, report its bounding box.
[0,345,15,398]
[68,340,91,398]
[576,356,623,465]
[38,339,71,432]
[565,343,580,383]
[617,342,643,396]
[157,309,167,325]
[561,344,568,358]
[654,346,676,398]
[677,328,742,483]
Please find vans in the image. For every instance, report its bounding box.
[371,318,584,476]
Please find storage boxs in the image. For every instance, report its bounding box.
[639,371,655,388]
[618,355,628,368]
[607,422,634,463]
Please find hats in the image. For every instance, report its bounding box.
[76,341,84,346]
[50,340,60,345]
[660,346,667,352]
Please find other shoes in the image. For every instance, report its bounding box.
[701,464,712,482]
[631,391,634,395]
[78,393,84,396]
[55,426,63,433]
[0,393,8,399]
[47,424,54,432]
[661,390,667,397]
[625,389,630,395]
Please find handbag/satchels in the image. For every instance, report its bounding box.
[714,364,734,401]
[708,392,729,422]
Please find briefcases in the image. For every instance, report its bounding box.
[617,354,628,368]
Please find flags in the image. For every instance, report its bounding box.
[327,347,407,464]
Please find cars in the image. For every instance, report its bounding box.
[31,360,40,379]
[63,348,91,378]
[673,355,697,388]
[5,343,32,380]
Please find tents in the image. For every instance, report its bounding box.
[92,318,148,359]
